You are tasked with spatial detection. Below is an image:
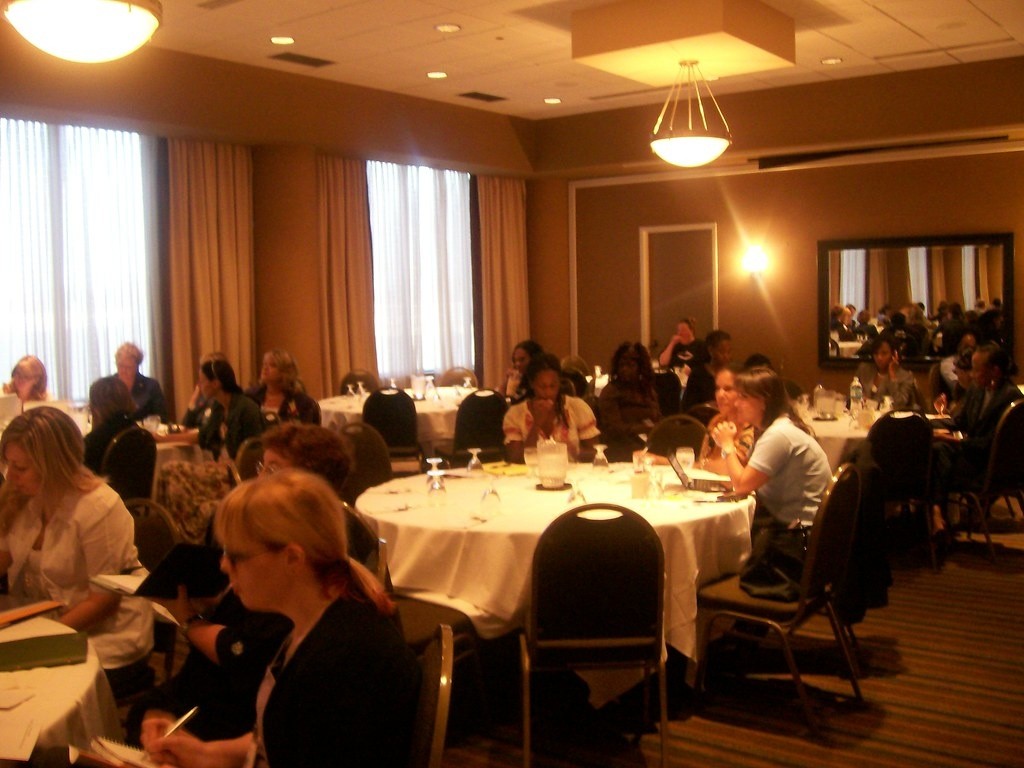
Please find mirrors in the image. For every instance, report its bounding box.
[817,231,1017,380]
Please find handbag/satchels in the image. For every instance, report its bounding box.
[740,516,813,597]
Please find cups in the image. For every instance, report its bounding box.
[633,450,651,474]
[537,443,569,488]
[933,399,944,416]
[676,448,695,475]
[815,391,893,427]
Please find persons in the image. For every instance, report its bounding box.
[711,366,833,542]
[0,355,52,436]
[855,335,914,411]
[140,469,423,768]
[83,376,141,473]
[0,406,154,697]
[928,325,1024,538]
[181,349,322,461]
[830,298,1004,358]
[594,340,665,462]
[126,424,352,750]
[89,342,170,423]
[698,365,753,474]
[502,339,601,463]
[659,316,772,404]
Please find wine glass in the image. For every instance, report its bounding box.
[523,448,538,479]
[345,375,473,402]
[479,473,503,511]
[593,444,609,471]
[467,448,485,476]
[423,455,449,506]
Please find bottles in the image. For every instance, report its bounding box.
[849,376,863,412]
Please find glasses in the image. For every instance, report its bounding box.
[225,547,277,568]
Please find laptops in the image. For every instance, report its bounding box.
[666,450,734,492]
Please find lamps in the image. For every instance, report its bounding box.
[572,0,795,171]
[1,0,163,64]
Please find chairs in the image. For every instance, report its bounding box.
[100,366,1024,768]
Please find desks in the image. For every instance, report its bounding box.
[0,616,126,754]
[73,412,205,500]
[355,461,758,726]
[800,407,953,483]
[315,386,478,450]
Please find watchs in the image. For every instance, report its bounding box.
[721,446,737,460]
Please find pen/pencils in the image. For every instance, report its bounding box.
[137,704,201,761]
[695,500,731,503]
